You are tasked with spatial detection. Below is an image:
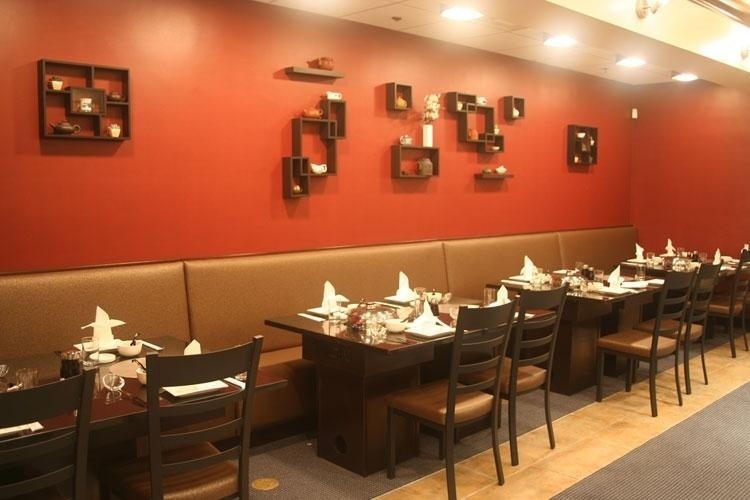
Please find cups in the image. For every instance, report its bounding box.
[79,97,92,113]
[633,264,647,281]
[676,247,686,258]
[60,348,81,377]
[359,310,390,339]
[102,375,126,405]
[15,366,39,390]
[646,252,657,266]
[484,287,497,307]
[592,270,604,283]
[108,129,120,137]
[81,336,100,366]
[552,270,562,287]
[400,136,413,145]
[49,80,63,90]
[660,254,675,270]
[675,259,690,273]
[326,298,342,325]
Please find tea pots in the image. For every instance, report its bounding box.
[106,90,126,101]
[316,57,333,70]
[49,118,82,135]
[307,163,328,177]
[395,97,408,110]
[455,93,508,176]
[416,155,433,176]
[325,90,344,101]
[301,106,324,120]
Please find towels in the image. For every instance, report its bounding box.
[182,337,204,356]
[395,268,415,300]
[87,304,117,346]
[411,300,449,334]
[319,276,342,313]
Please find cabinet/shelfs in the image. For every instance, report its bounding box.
[384,82,440,179]
[280,66,346,200]
[565,122,600,167]
[35,56,131,144]
[448,90,525,181]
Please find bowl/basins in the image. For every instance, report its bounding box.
[135,367,149,387]
[114,340,145,357]
[576,133,585,138]
[426,292,443,304]
[384,317,401,334]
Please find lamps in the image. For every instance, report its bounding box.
[634,0,662,20]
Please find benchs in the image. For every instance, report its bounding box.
[184,240,449,436]
[1,256,238,443]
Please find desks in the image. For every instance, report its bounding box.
[485,273,665,398]
[631,259,724,395]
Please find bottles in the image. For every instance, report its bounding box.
[582,264,594,283]
[687,251,698,262]
[417,296,439,318]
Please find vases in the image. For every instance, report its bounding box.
[420,123,435,147]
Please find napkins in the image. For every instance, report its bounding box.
[520,254,539,278]
[665,238,676,254]
[741,244,749,254]
[712,247,722,265]
[635,242,645,258]
[607,265,625,291]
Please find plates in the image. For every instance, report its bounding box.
[381,294,418,304]
[648,278,664,286]
[508,275,531,283]
[404,325,452,338]
[71,339,118,352]
[160,378,231,399]
[304,305,346,318]
[89,353,116,364]
[511,311,537,324]
[597,284,628,295]
[626,259,658,264]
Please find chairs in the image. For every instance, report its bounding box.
[456,279,572,468]
[0,366,98,500]
[381,292,522,500]
[707,248,749,359]
[94,332,265,500]
[595,266,699,416]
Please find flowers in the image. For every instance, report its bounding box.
[418,92,444,123]
